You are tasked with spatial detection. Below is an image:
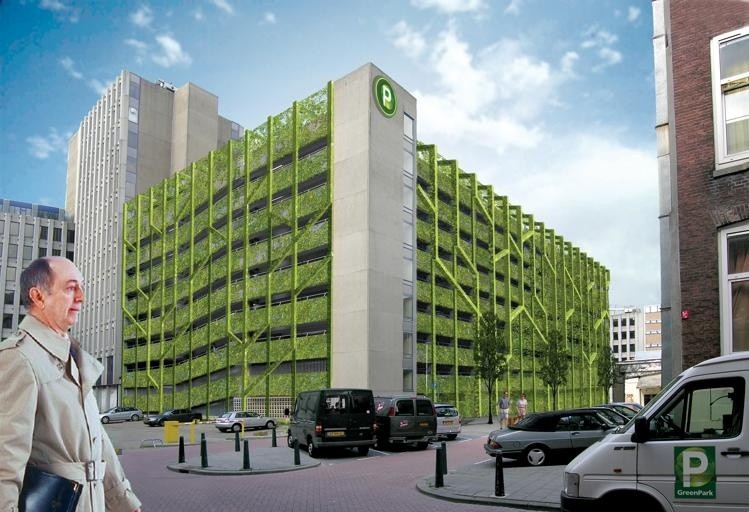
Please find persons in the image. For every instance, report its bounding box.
[499,391,510,430]
[515,393,528,422]
[0,255,142,511]
